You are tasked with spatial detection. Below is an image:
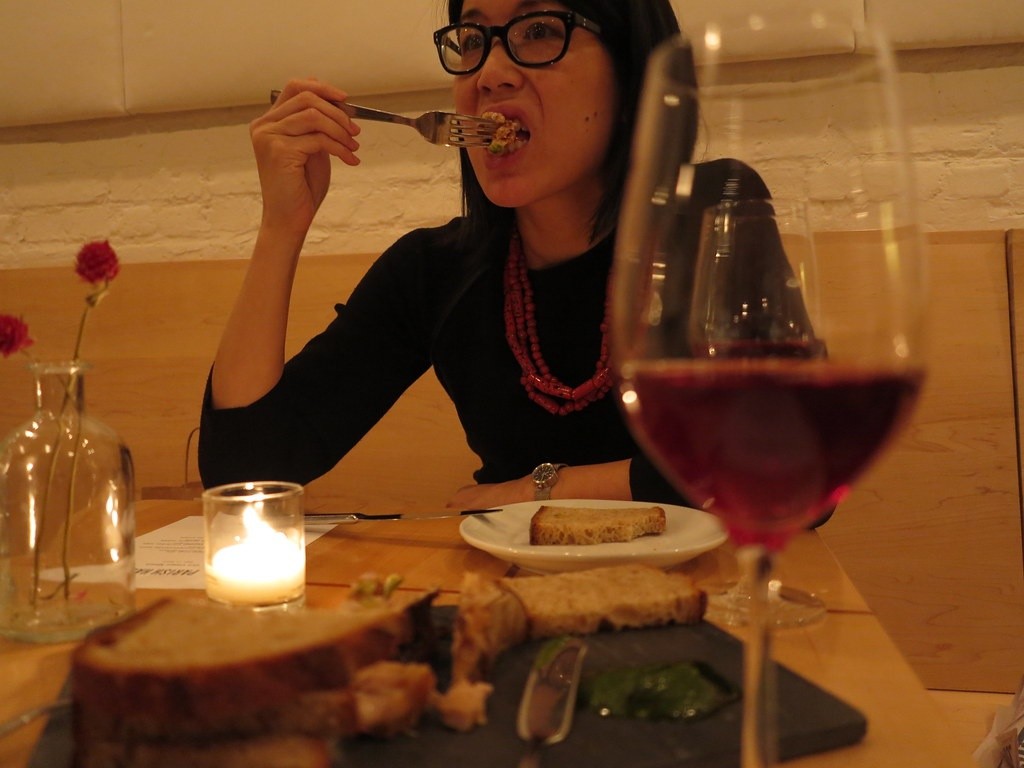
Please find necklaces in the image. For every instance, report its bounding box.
[503,232,655,416]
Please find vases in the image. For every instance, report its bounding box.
[0,357,137,645]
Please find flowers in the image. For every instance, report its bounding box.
[0,237,123,615]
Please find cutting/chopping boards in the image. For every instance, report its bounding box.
[26,618,867,768]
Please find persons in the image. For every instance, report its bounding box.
[198,0,839,530]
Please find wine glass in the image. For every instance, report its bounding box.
[687,199,825,629]
[606,8,928,765]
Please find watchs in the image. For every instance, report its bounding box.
[531,462,569,500]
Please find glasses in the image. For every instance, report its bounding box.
[433,9,606,75]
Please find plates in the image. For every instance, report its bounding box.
[459,498,727,575]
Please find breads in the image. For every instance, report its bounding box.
[529,505,666,546]
[59,596,429,768]
[434,562,706,728]
[481,112,519,154]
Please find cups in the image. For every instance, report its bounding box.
[203,482,306,613]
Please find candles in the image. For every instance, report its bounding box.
[200,480,305,605]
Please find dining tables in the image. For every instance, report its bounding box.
[0,486,981,768]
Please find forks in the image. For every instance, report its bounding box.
[270,89,502,148]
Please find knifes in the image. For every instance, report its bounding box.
[304,509,503,523]
